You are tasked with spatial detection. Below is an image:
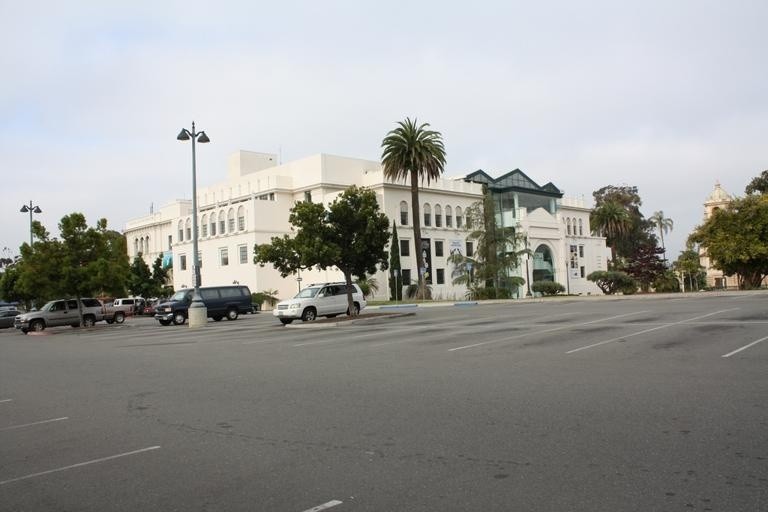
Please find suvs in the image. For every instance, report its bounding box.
[13,296,107,334]
[271,281,369,325]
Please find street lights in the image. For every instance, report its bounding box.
[564,256,569,295]
[19,199,45,246]
[521,230,532,298]
[177,120,213,329]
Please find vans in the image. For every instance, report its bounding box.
[155,284,252,327]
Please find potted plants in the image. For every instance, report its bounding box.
[251,286,284,311]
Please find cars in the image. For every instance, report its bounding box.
[0,303,21,330]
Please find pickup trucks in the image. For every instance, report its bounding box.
[97,296,167,325]
